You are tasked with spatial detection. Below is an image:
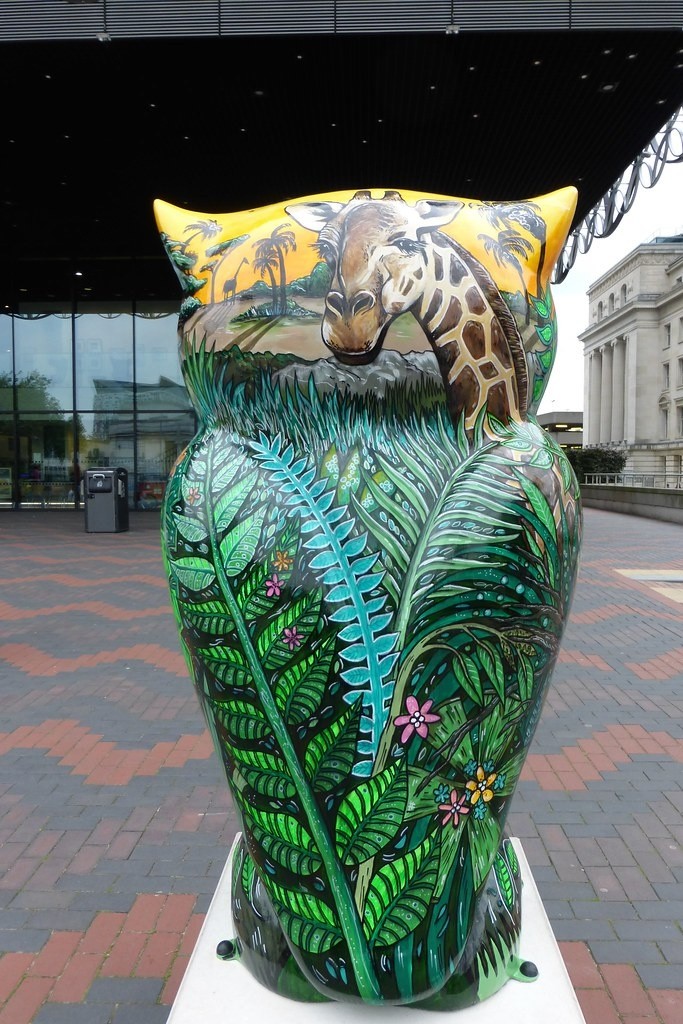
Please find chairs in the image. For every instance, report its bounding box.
[49,483,67,506]
[20,484,32,508]
[29,485,47,506]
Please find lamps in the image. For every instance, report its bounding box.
[97,33,111,43]
[445,25,460,34]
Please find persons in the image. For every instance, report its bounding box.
[29,464,40,480]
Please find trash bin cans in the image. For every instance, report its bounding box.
[84,467,128,533]
